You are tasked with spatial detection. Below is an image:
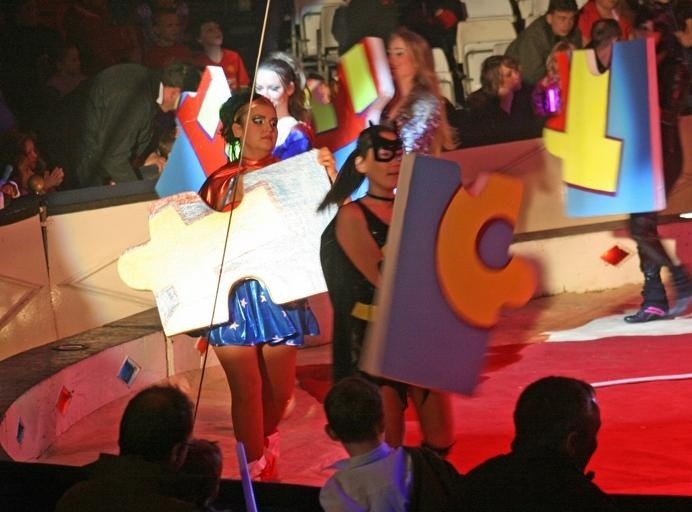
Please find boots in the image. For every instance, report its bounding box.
[668,265,692,316]
[625,262,669,322]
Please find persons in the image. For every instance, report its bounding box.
[587,19,692,321]
[1,1,691,211]
[334,128,455,464]
[450,376,619,509]
[180,95,350,483]
[52,385,221,512]
[317,376,459,512]
[173,439,222,512]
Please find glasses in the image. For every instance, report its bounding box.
[369,121,403,162]
